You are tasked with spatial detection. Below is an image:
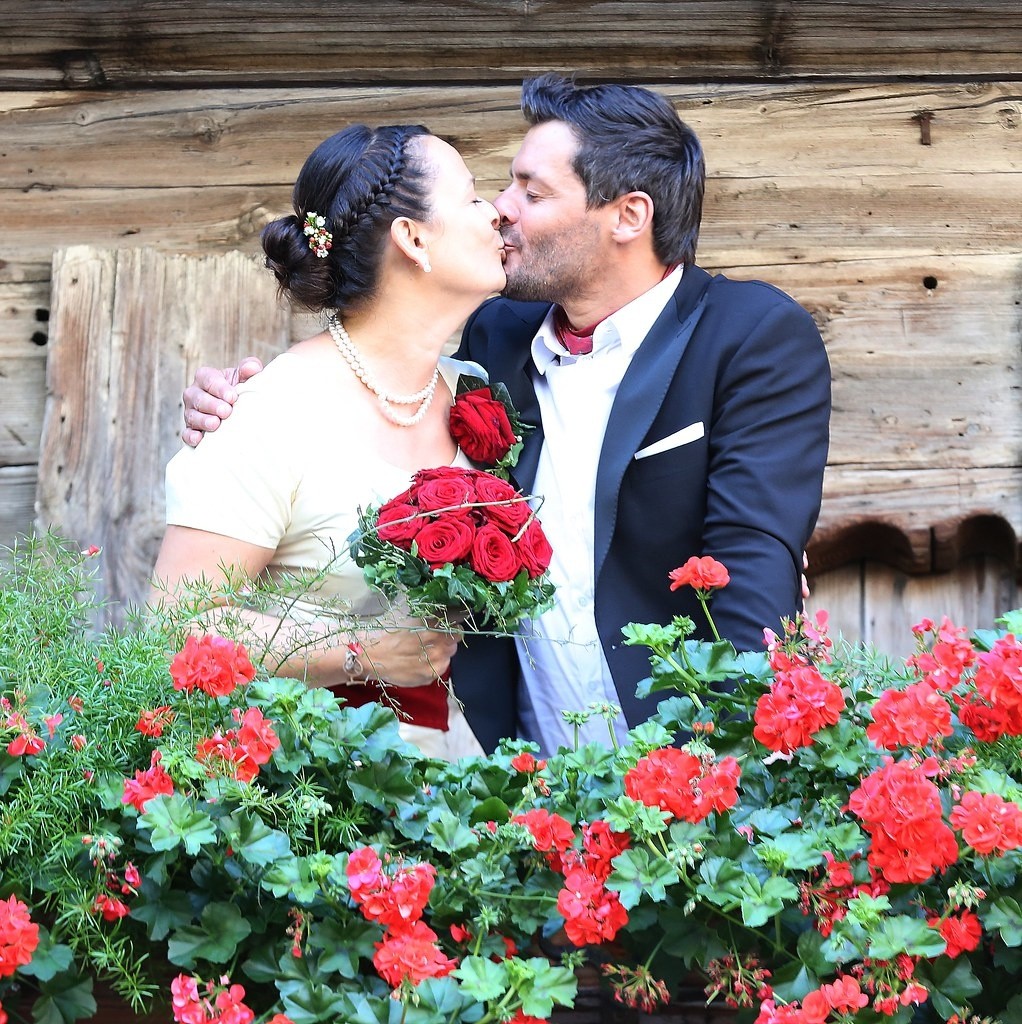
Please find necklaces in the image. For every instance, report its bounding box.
[328,312,439,427]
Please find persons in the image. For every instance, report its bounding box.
[146,124,811,762]
[182,70,832,805]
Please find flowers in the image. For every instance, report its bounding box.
[449,373,538,481]
[346,466,557,634]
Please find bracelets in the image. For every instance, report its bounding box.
[344,640,367,685]
